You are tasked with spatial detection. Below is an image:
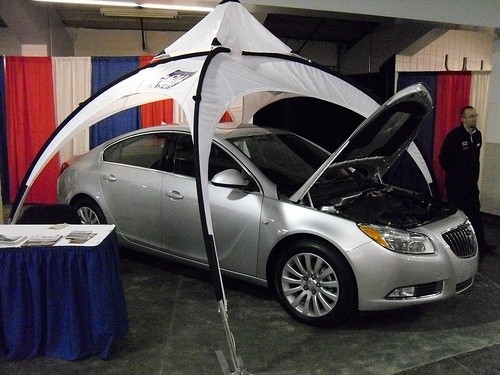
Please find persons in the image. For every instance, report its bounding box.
[442,97,492,259]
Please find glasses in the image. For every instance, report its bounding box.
[465,114,479,119]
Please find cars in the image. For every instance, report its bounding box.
[56,80,480,328]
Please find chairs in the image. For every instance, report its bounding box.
[18,204,81,224]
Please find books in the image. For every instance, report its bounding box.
[1,224,98,249]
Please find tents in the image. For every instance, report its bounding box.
[11,1,431,368]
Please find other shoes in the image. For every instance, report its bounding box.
[479,239,498,253]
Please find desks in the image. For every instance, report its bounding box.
[0,222,131,363]
